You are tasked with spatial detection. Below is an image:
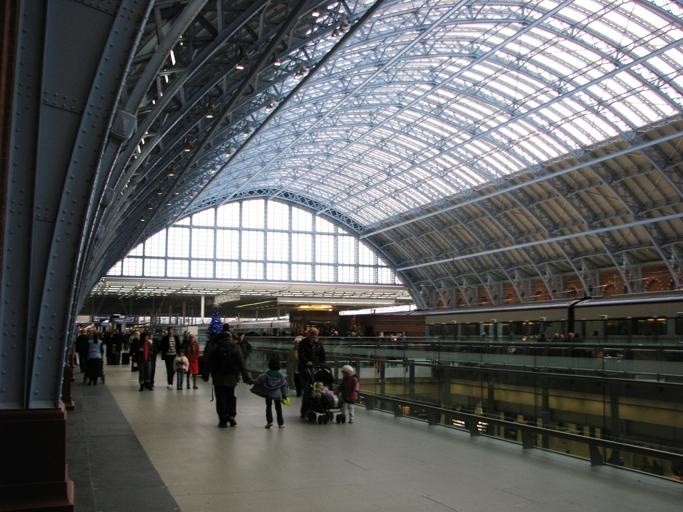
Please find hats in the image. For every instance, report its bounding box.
[340,364,355,375]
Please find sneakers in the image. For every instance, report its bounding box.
[265,421,274,430]
[278,423,286,429]
[217,419,229,428]
[228,416,237,427]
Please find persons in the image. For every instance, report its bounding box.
[480,332,488,353]
[651,458,660,474]
[75,324,359,428]
[537,331,600,357]
[640,456,652,472]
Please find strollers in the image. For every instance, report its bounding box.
[303,364,346,425]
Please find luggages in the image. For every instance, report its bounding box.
[121,352,130,364]
